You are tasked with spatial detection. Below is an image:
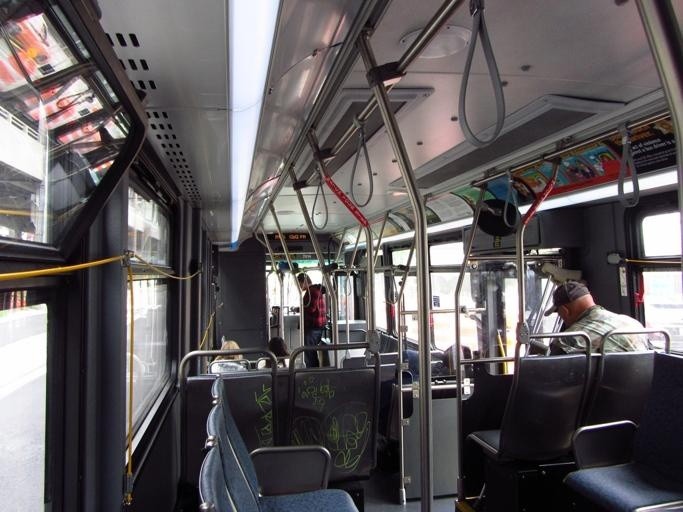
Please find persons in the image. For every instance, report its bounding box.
[292,273,328,368]
[212,340,247,372]
[542,281,654,353]
[258,336,289,369]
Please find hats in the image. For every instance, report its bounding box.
[264,338,288,357]
[544,281,591,317]
[220,341,243,360]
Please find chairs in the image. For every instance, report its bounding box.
[367,325,681,508]
[182,325,393,512]
